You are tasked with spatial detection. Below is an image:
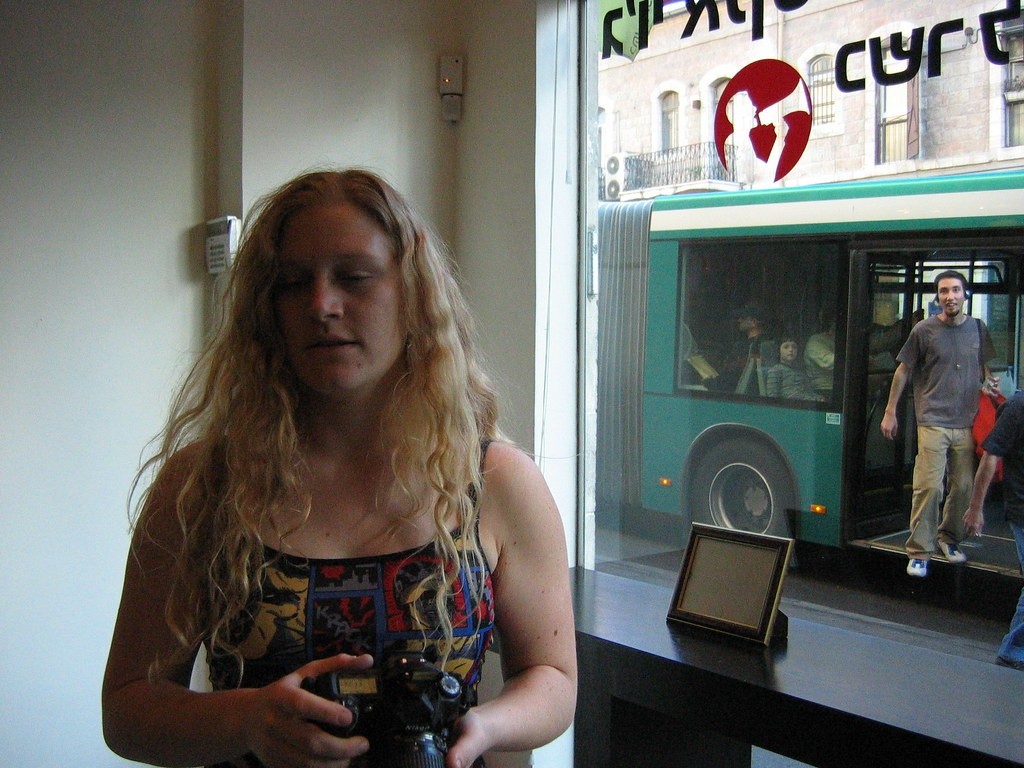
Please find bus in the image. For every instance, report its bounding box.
[593,164,1024,578]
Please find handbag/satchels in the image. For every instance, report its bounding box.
[975,392,1007,482]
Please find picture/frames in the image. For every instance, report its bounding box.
[668,520,797,649]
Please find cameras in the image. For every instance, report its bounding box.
[300,650,478,768]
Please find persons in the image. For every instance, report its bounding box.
[694,268,846,404]
[961,389,1024,671]
[101,170,580,768]
[879,270,998,578]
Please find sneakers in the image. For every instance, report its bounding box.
[997,653,1024,670]
[906,558,926,578]
[936,539,967,563]
